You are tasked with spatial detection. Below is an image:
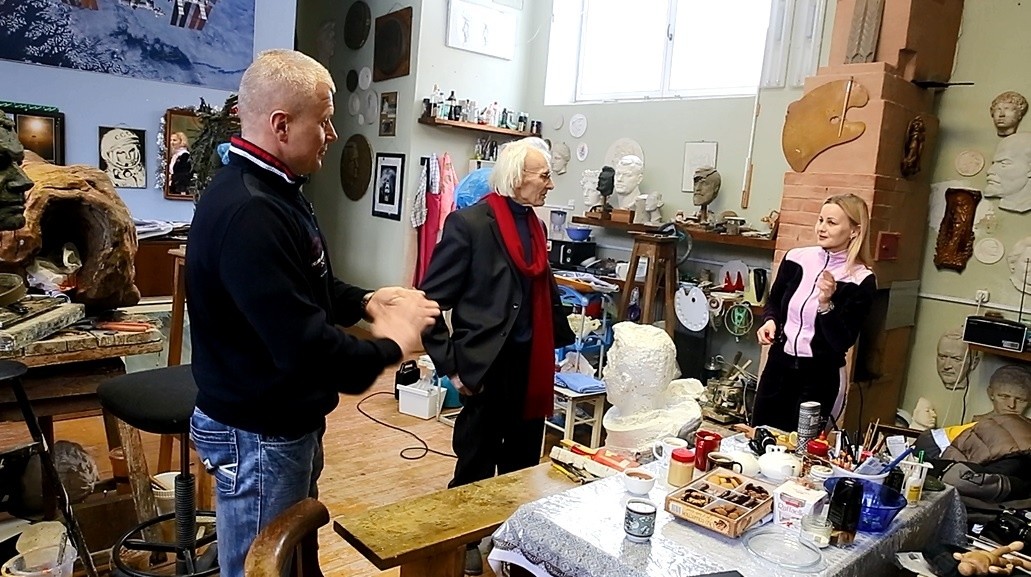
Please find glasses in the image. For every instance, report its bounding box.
[522,168,553,182]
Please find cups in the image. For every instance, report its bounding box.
[730,452,759,478]
[151,471,181,516]
[0,542,78,577]
[694,430,722,472]
[623,468,656,495]
[549,210,567,241]
[623,498,657,543]
[652,437,688,468]
[828,462,891,486]
[798,514,832,549]
[705,452,743,474]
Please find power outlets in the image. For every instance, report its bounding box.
[976,291,989,302]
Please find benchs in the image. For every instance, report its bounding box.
[334,459,578,577]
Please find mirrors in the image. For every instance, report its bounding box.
[164,108,202,201]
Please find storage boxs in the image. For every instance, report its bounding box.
[664,465,781,539]
[773,480,829,534]
[396,380,447,420]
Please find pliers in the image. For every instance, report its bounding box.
[75,321,151,332]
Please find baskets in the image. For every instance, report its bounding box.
[823,475,908,534]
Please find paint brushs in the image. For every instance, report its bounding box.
[831,417,889,473]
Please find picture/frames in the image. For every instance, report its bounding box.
[371,152,405,221]
[0,101,64,166]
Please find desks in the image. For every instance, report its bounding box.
[488,425,966,577]
[617,234,677,340]
[0,319,166,577]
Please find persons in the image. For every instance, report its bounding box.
[99,129,146,188]
[598,321,705,452]
[0,108,35,231]
[579,169,614,218]
[936,330,981,390]
[692,165,722,206]
[909,397,937,431]
[645,192,664,221]
[752,192,878,439]
[416,138,576,576]
[984,132,1031,213]
[613,155,644,209]
[596,165,616,197]
[551,143,571,175]
[1006,237,1031,295]
[382,97,390,133]
[987,92,1028,137]
[972,364,1031,423]
[182,48,440,577]
[169,131,192,194]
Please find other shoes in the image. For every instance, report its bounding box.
[464,546,483,575]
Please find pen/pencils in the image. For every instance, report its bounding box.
[878,446,916,476]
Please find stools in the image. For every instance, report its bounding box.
[96,364,220,577]
[540,389,608,457]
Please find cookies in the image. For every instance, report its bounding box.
[681,474,769,518]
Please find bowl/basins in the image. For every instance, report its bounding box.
[822,476,907,532]
[565,221,593,242]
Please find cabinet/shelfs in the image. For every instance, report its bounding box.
[570,217,778,317]
[559,284,612,379]
[135,238,187,296]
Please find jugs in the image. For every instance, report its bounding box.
[758,444,801,481]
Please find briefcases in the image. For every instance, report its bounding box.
[395,360,420,400]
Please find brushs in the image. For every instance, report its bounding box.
[550,461,581,483]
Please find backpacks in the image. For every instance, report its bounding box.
[429,368,463,408]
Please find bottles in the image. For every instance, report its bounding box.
[667,448,695,488]
[825,478,863,548]
[805,466,833,491]
[814,431,828,445]
[904,471,923,508]
[422,84,541,135]
[801,440,830,477]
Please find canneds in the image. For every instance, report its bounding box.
[668,448,695,487]
[800,440,829,477]
